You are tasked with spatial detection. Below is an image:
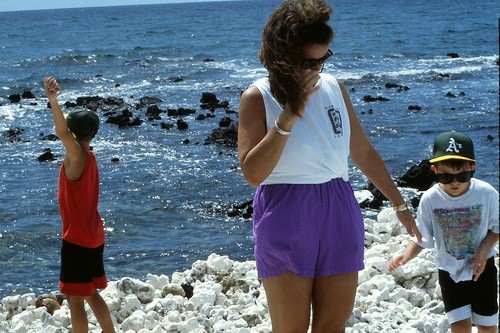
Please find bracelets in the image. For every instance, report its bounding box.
[273,120,292,136]
[391,201,410,213]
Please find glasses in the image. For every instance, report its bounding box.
[297,49,333,70]
[436,170,475,184]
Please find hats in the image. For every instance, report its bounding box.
[63,109,99,140]
[429,130,476,163]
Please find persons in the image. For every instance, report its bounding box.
[236,0,423,333]
[388,130,500,333]
[45,76,118,333]
